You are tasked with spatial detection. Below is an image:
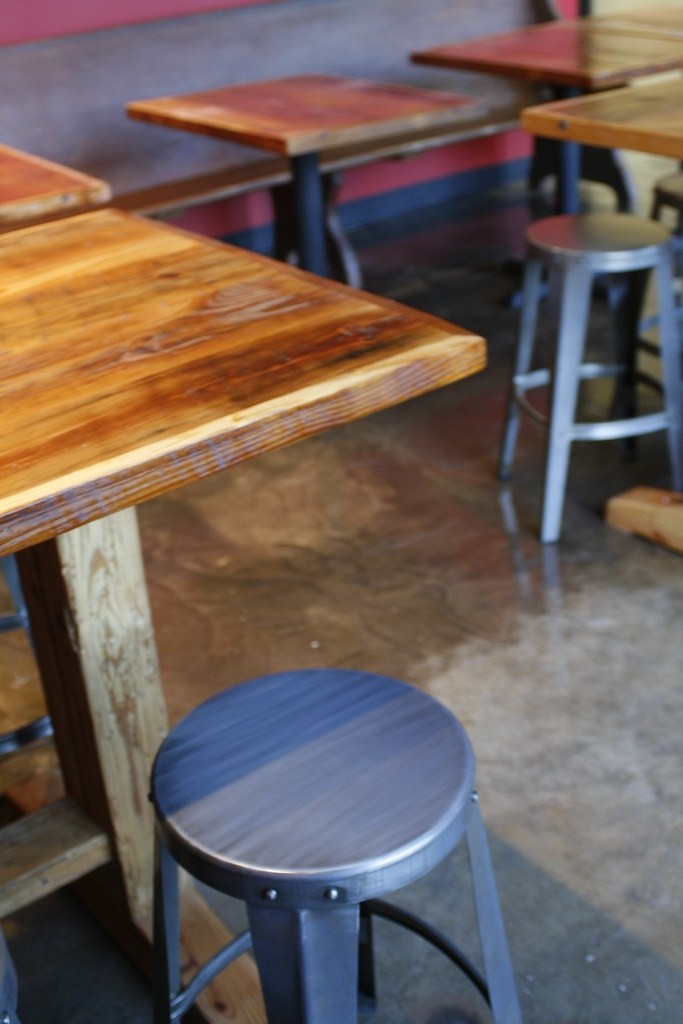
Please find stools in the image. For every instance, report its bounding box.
[637,171,683,357]
[148,669,526,1023]
[500,217,681,543]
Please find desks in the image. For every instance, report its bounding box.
[0,206,486,1024]
[409,0,683,552]
[0,144,113,234]
[125,73,489,281]
